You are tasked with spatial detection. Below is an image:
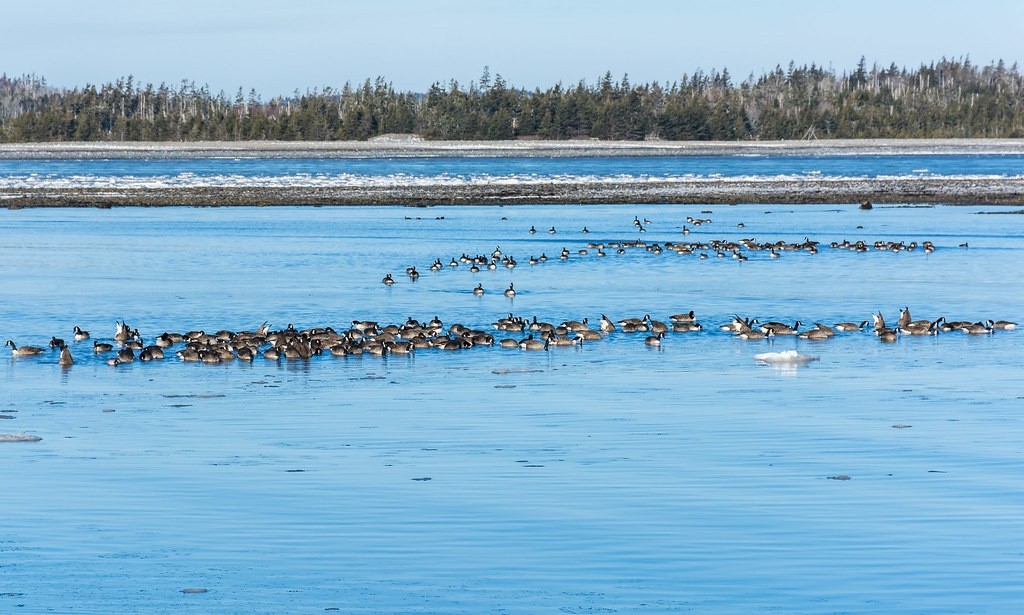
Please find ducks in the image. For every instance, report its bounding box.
[3,216,1018,368]
